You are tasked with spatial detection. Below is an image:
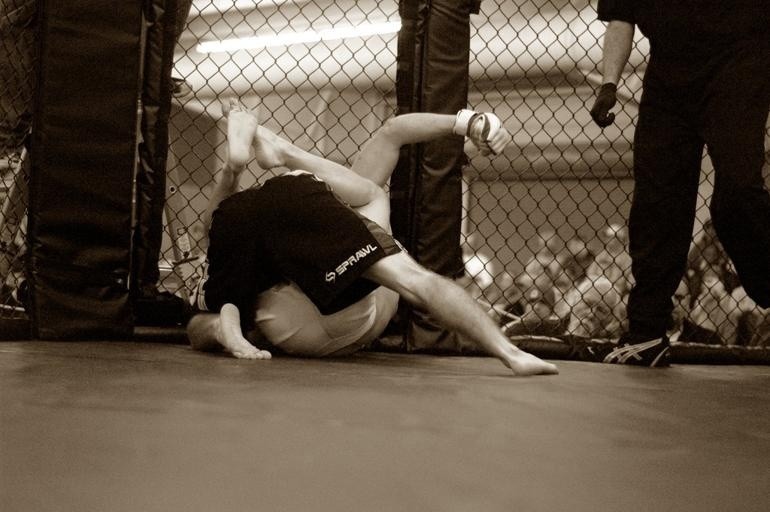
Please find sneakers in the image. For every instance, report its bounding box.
[582,330,675,370]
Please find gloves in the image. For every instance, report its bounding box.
[450,107,515,159]
[588,80,619,128]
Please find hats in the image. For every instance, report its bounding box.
[524,283,557,316]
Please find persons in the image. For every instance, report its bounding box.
[185,106,564,380]
[201,107,401,359]
[588,1,769,370]
